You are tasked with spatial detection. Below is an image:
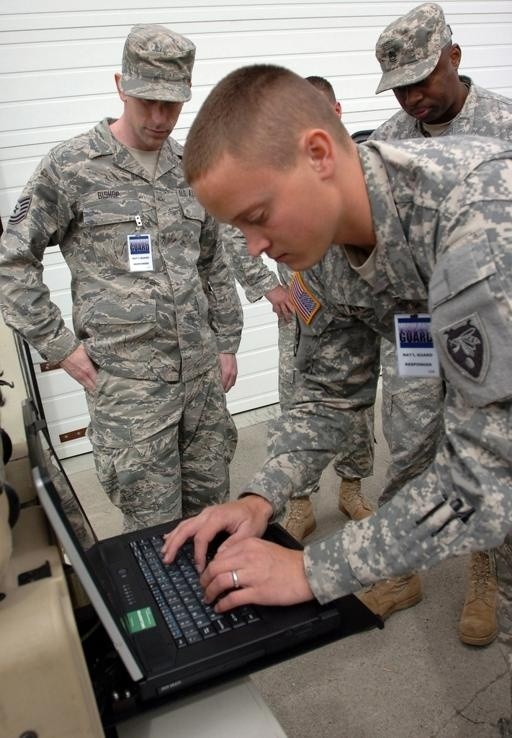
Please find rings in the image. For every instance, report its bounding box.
[231,570,239,590]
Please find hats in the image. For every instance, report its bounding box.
[121,23,197,103]
[375,3,453,94]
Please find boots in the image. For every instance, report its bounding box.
[280,495,316,544]
[458,551,499,646]
[338,475,375,522]
[357,567,424,621]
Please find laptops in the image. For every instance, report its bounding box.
[22,397,343,703]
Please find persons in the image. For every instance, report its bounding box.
[352,3,511,646]
[157,66,511,652]
[1,24,245,536]
[221,74,377,542]
